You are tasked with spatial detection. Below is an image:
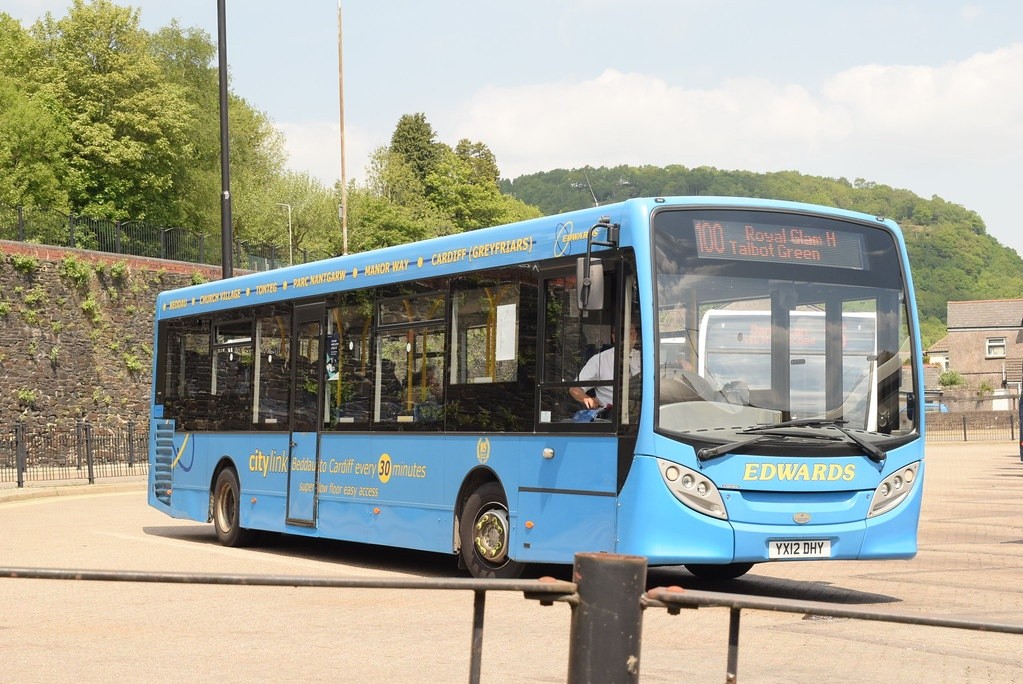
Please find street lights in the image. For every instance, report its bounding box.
[275,203,293,268]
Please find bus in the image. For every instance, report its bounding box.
[660,337,689,375]
[147,196,927,578]
[697,309,878,431]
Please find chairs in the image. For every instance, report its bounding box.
[182,349,442,423]
[556,334,595,414]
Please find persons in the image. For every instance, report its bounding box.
[568,318,695,413]
[343,363,442,426]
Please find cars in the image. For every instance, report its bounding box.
[899,404,951,413]
